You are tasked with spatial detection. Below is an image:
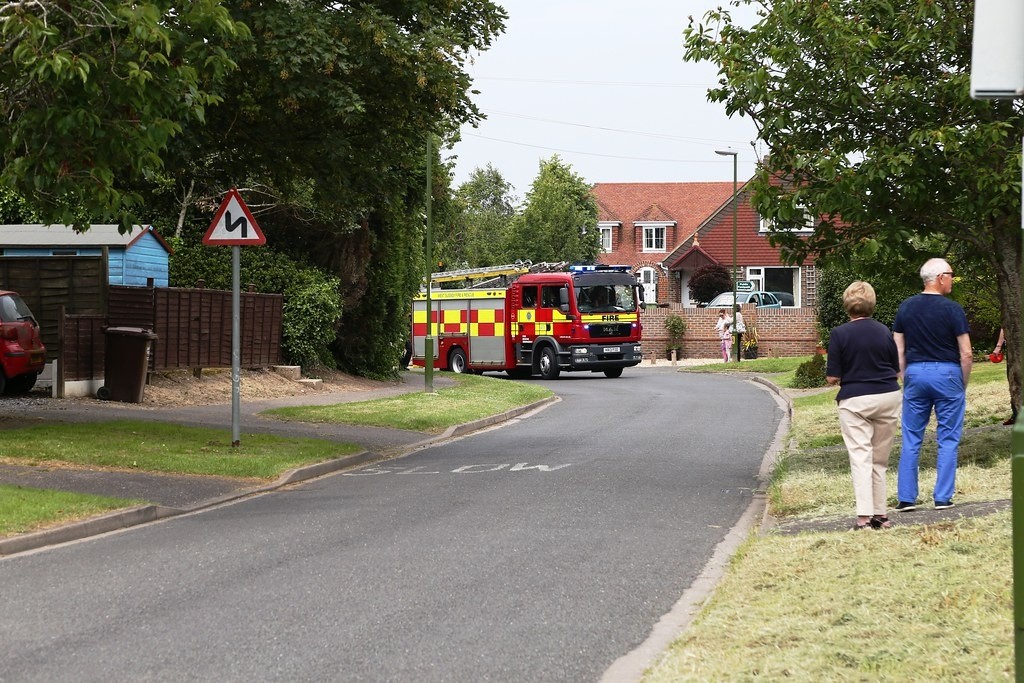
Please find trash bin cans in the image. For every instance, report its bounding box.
[97,324,158,403]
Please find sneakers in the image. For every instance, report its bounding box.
[893,501,916,512]
[934,501,955,509]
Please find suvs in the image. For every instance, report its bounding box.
[0,290,47,399]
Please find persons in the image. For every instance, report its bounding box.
[721,322,733,363]
[826,280,901,531]
[892,258,972,511]
[992,324,1018,426]
[729,304,746,362]
[713,309,733,362]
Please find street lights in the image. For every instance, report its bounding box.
[716,149,740,360]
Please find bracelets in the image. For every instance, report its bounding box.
[996,345,1002,347]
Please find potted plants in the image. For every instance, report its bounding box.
[741,326,759,360]
[664,314,687,361]
[816,341,828,355]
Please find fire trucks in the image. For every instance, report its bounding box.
[413,258,645,382]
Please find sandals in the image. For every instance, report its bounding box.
[845,523,875,532]
[868,516,891,530]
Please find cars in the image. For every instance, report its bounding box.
[702,289,781,308]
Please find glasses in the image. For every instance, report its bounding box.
[719,315,723,317]
[937,271,956,278]
